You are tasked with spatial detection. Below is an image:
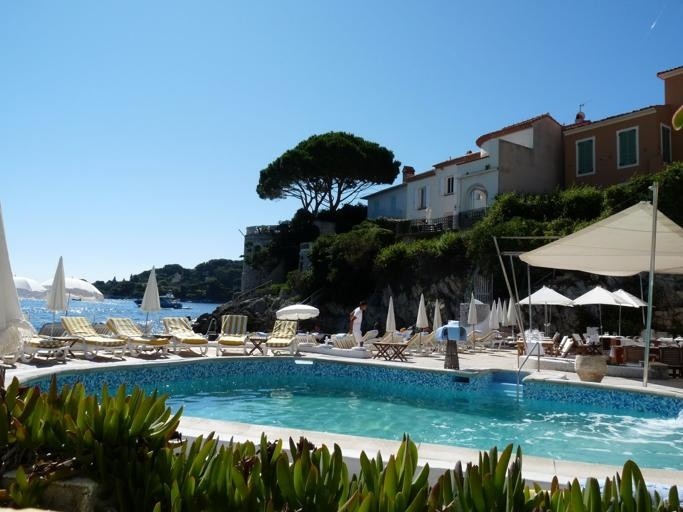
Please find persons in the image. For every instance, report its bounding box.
[348,300,367,347]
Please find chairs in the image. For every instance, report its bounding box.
[514,326,683,378]
[372,329,503,363]
[16,313,301,365]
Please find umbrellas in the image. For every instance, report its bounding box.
[432,297,442,353]
[385,295,395,343]
[276,302,319,332]
[10,275,43,302]
[415,292,428,351]
[44,255,67,334]
[0,207,21,368]
[466,286,656,352]
[40,275,103,316]
[136,264,162,331]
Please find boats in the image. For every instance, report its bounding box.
[134,289,182,310]
